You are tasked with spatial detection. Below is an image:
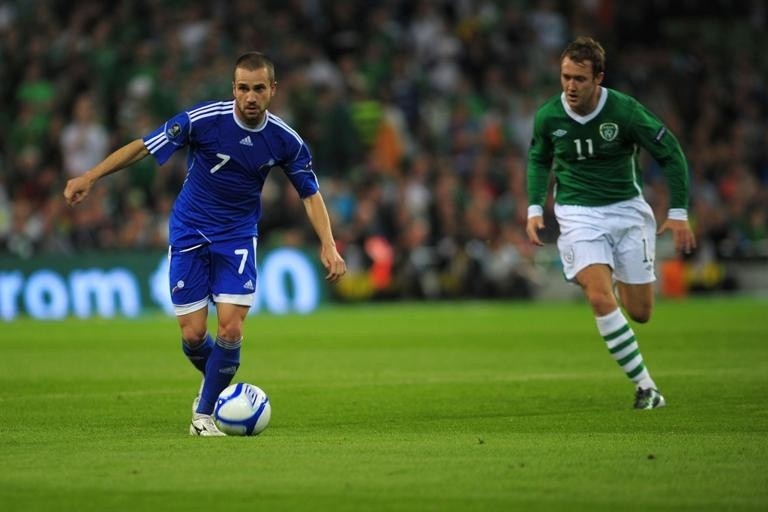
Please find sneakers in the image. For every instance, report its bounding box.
[190,376,226,436]
[634,388,665,409]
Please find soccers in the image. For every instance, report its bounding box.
[212,384,272,436]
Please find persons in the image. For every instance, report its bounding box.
[526,38,697,409]
[63,51,349,444]
[2,1,767,297]
